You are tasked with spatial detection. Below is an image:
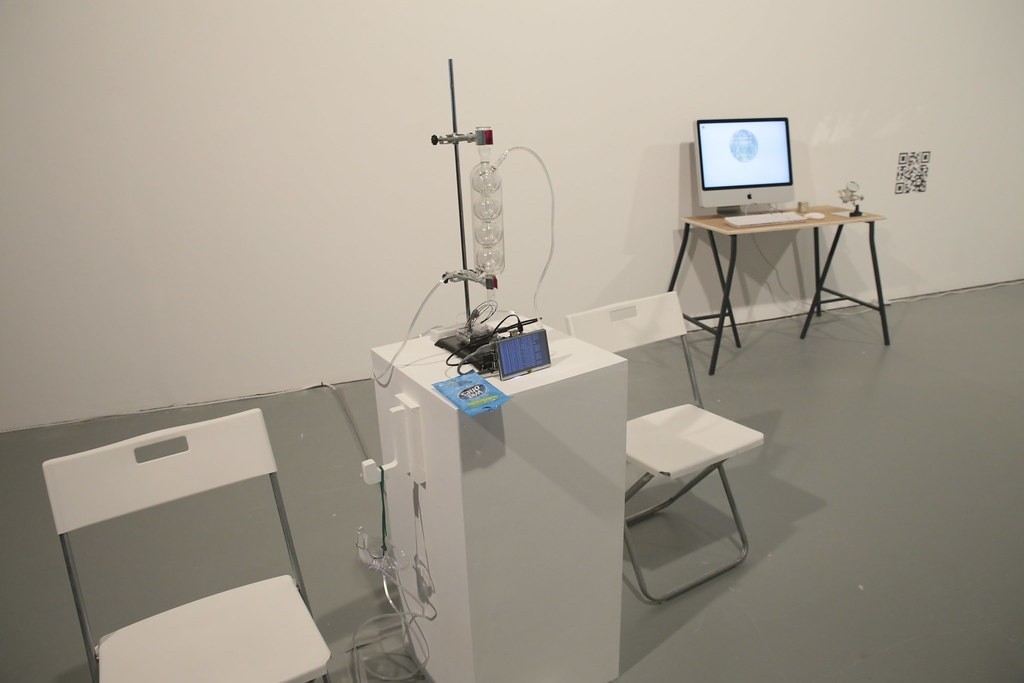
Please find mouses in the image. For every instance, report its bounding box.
[805,212,826,220]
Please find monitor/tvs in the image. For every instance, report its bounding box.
[695,117,795,217]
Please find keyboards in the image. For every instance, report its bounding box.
[724,211,806,228]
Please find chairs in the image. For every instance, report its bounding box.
[42,406,333,683]
[566,290,764,604]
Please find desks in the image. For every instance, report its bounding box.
[668,204,891,375]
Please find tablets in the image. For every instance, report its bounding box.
[494,329,551,381]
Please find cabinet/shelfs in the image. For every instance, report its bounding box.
[371,318,627,683]
